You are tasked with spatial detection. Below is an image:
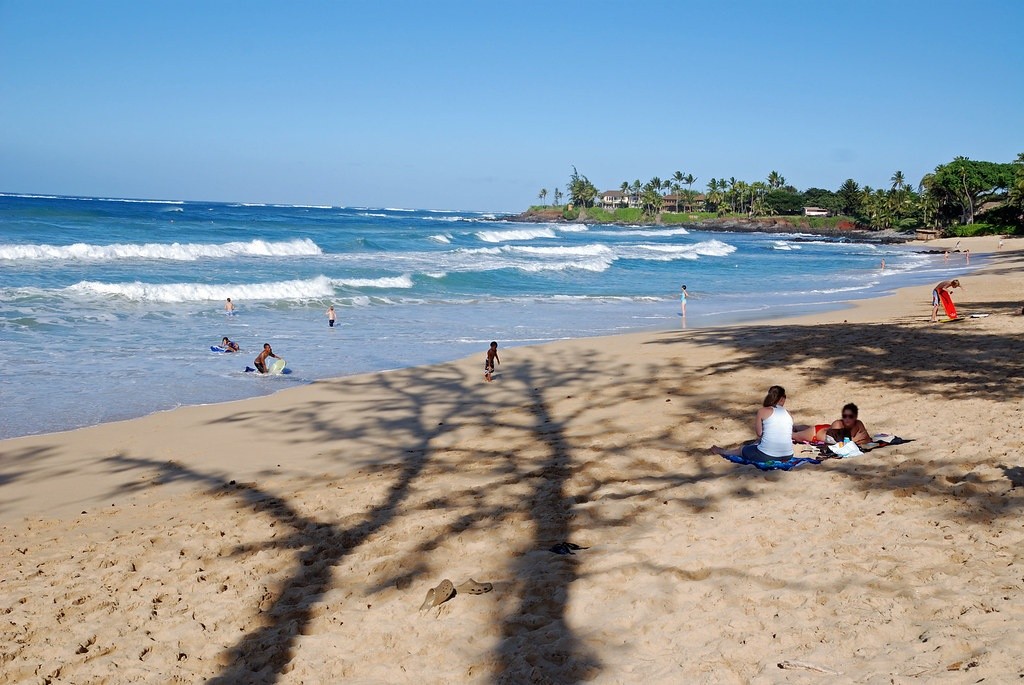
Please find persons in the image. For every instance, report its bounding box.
[931,279,962,323]
[882,237,1003,268]
[711,386,794,462]
[485,341,500,382]
[254,344,281,375]
[222,298,240,352]
[327,306,336,328]
[792,403,871,446]
[681,285,689,317]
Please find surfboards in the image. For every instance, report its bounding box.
[268,359,285,375]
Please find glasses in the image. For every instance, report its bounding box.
[843,414,854,418]
[783,395,786,399]
